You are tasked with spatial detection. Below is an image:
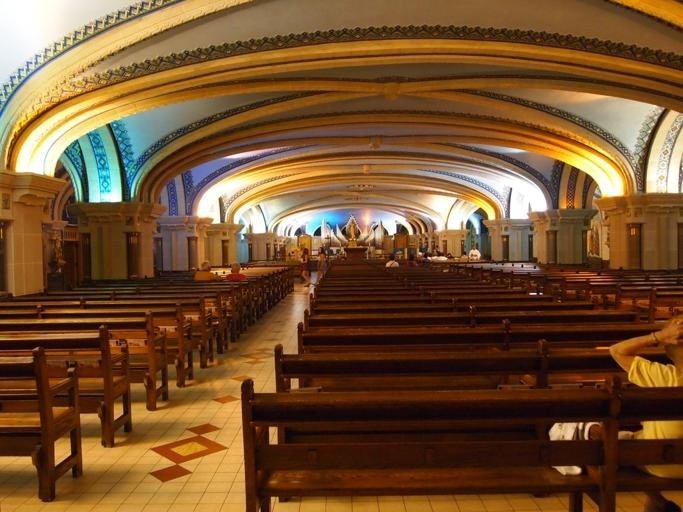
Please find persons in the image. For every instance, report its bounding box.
[384,252,399,268]
[606,307,682,511]
[192,261,222,281]
[458,251,468,262]
[299,248,312,287]
[415,244,455,272]
[311,247,326,286]
[468,244,481,262]
[224,261,247,281]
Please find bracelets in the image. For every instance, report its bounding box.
[649,331,659,347]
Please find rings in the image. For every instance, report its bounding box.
[676,320,680,325]
